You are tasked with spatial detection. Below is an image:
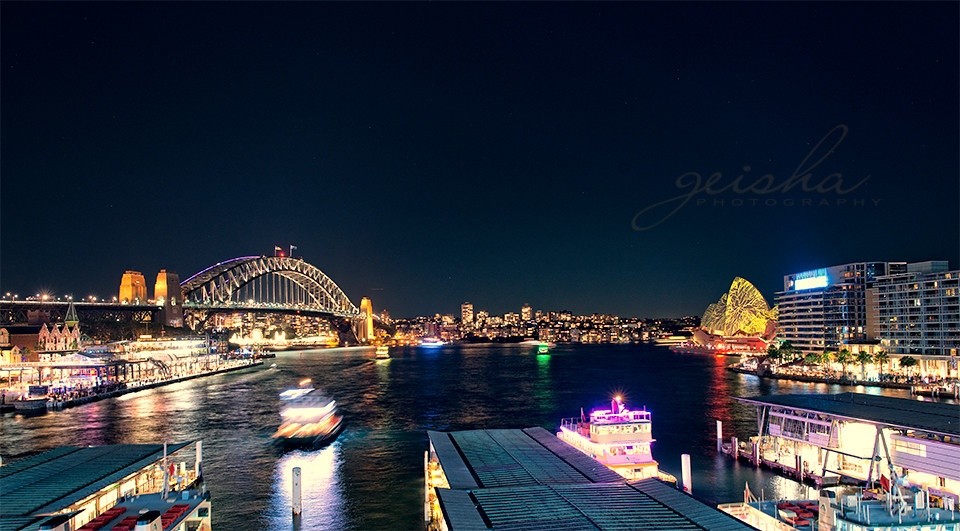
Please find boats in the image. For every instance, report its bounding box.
[537,344,550,354]
[226,344,276,359]
[375,344,392,360]
[555,392,677,486]
[274,387,345,446]
[417,341,443,349]
[12,398,49,415]
